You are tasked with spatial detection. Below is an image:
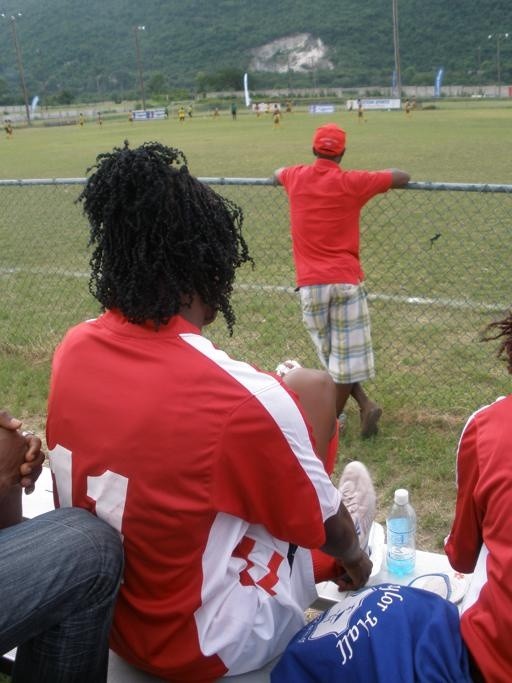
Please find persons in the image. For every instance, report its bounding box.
[79,112,84,127]
[97,111,102,128]
[178,106,185,122]
[188,104,193,118]
[212,106,220,120]
[40,134,380,682]
[129,109,135,123]
[271,102,282,130]
[356,97,368,123]
[272,121,412,441]
[254,102,260,118]
[0,410,128,682]
[404,98,412,116]
[439,307,512,680]
[4,111,14,139]
[231,101,237,120]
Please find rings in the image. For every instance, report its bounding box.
[22,429,35,437]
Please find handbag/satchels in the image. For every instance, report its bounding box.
[272,581,472,683]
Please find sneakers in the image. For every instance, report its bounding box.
[339,459,375,559]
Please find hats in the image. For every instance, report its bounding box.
[313,122,346,158]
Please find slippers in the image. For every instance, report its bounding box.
[338,405,382,438]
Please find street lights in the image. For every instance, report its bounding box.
[0,12,32,126]
[488,32,509,96]
[133,24,146,111]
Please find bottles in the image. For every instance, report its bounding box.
[386,489,416,574]
